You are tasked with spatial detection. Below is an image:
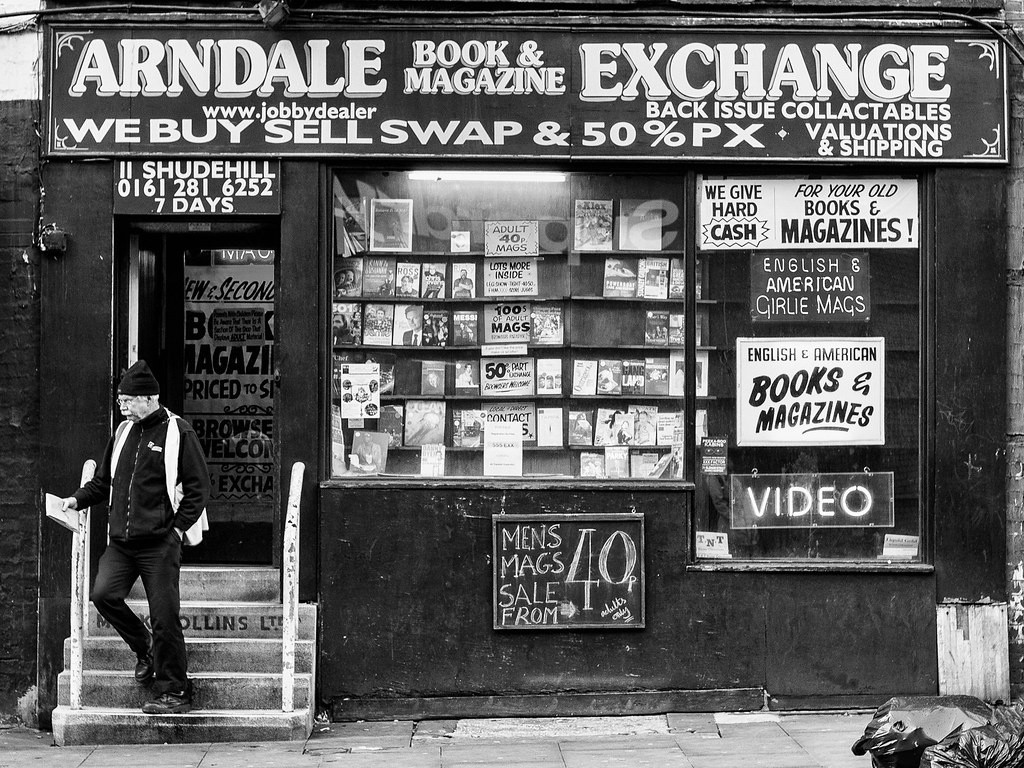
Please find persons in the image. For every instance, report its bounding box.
[708,454,762,563]
[58,361,212,715]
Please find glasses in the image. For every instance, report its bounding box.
[116,397,140,406]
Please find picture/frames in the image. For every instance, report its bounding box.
[370,199,414,252]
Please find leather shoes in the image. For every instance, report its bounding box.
[142,690,192,714]
[135,632,157,682]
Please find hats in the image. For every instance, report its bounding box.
[117,360,160,395]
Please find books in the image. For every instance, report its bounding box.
[326,197,707,480]
[44,493,80,532]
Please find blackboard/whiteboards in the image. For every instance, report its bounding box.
[493,513,645,629]
[751,252,870,324]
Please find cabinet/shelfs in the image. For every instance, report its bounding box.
[332,169,921,561]
